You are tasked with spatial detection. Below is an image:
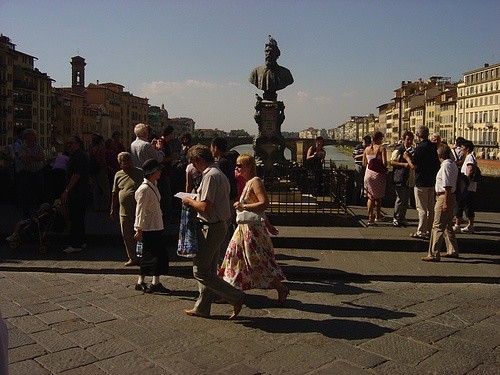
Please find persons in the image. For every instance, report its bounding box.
[248,36,294,92]
[109,151,144,267]
[133,159,167,291]
[216,152,291,306]
[419,143,460,262]
[181,143,246,320]
[1,121,480,254]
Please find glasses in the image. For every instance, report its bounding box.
[66,141,76,145]
[235,164,244,168]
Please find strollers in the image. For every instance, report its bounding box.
[3,199,67,254]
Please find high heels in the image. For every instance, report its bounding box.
[275,285,291,306]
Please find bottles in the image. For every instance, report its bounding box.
[136,241,144,258]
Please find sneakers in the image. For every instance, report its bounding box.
[137,282,151,293]
[149,282,171,295]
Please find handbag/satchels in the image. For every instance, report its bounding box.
[368,158,391,178]
[236,208,266,225]
[469,166,482,181]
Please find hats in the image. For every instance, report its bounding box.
[364,136,372,144]
[136,159,165,176]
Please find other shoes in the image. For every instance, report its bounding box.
[368,216,390,224]
[421,257,440,262]
[125,258,136,266]
[460,225,475,234]
[63,242,87,253]
[410,233,425,239]
[440,253,459,258]
[452,226,461,234]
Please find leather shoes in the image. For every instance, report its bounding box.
[229,292,249,319]
[185,308,211,319]
